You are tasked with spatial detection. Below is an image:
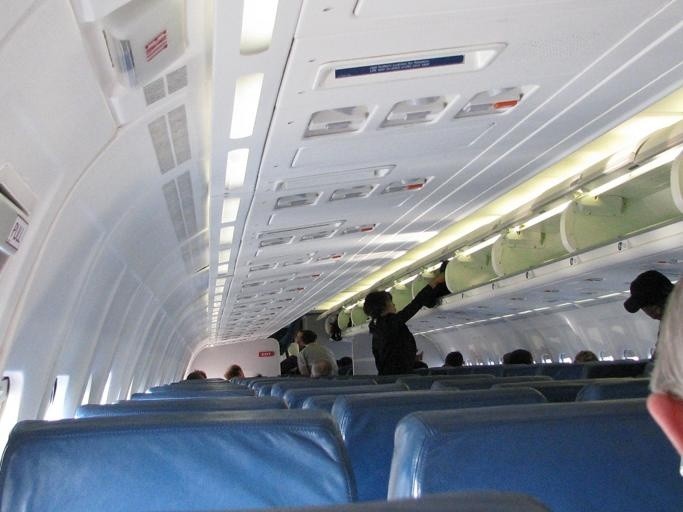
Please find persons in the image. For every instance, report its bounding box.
[364,271,444,375]
[646,276,683,473]
[574,350,599,362]
[623,269,672,321]
[224,364,244,380]
[187,370,207,379]
[503,349,533,366]
[278,328,351,379]
[444,351,464,367]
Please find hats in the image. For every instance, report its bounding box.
[624,270,671,313]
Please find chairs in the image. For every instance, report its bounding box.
[0,361,683,511]
